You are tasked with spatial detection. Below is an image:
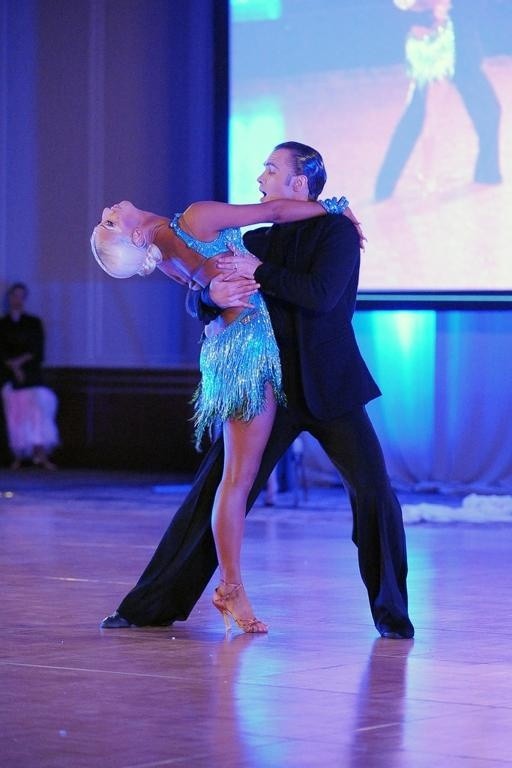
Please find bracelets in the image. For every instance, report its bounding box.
[317,196,349,216]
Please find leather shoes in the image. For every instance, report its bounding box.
[100,610,133,628]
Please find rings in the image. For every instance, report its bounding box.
[234,263,238,269]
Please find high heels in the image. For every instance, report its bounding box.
[212,579,270,634]
[32,456,58,472]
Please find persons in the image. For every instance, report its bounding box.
[100,142,414,640]
[89,196,368,635]
[0,282,61,470]
